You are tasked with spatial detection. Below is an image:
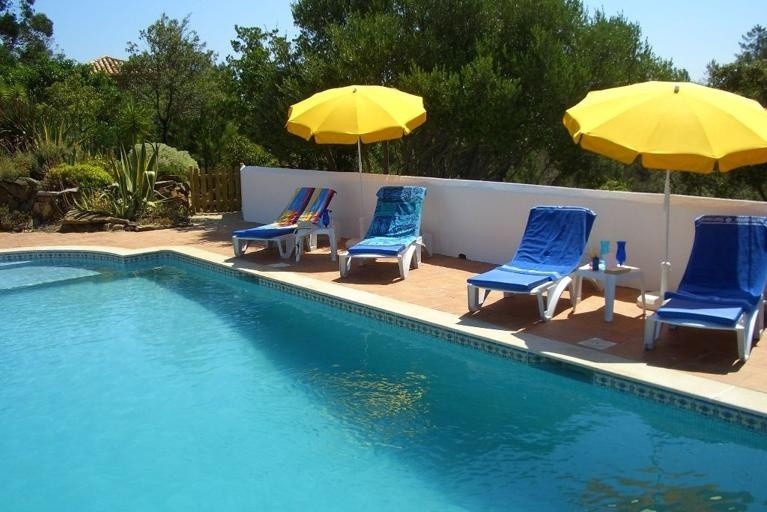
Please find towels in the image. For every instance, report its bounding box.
[665,216,767,314]
[495,206,597,281]
[357,186,428,248]
[276,186,337,227]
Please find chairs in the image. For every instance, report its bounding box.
[644,293,767,362]
[339,233,432,280]
[232,224,318,259]
[467,256,603,323]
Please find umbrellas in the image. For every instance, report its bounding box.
[282,84,431,243]
[560,80,767,302]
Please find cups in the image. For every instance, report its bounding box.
[592,240,616,271]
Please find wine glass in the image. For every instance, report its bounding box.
[322,209,329,227]
[616,241,627,265]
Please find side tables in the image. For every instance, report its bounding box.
[573,260,646,323]
[295,228,337,262]
